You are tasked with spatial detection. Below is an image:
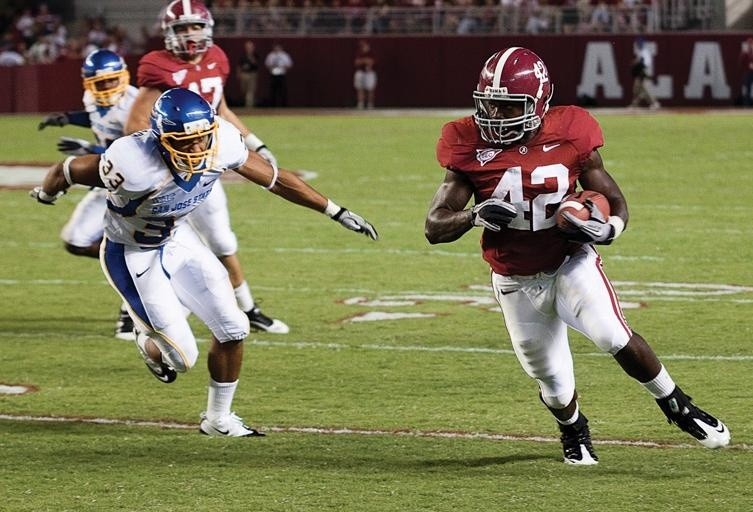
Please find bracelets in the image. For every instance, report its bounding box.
[38,190,56,202]
[322,198,341,217]
[243,132,265,151]
[608,214,625,239]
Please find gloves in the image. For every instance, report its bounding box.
[56,136,93,155]
[562,198,616,246]
[332,207,378,240]
[37,110,69,131]
[470,197,516,231]
[256,145,276,163]
[27,186,57,205]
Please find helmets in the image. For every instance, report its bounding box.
[81,49,130,80]
[474,47,556,123]
[160,0,215,26]
[151,88,218,153]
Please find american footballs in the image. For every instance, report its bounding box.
[557,191,610,234]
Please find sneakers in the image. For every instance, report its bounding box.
[134,328,177,384]
[116,308,136,341]
[243,306,290,333]
[559,425,599,467]
[672,404,731,449]
[199,414,263,437]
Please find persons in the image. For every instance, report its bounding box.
[27,88,379,438]
[423,46,732,467]
[113,0,290,342]
[738,39,753,107]
[35,49,140,261]
[350,39,377,109]
[205,1,753,39]
[0,0,131,65]
[234,39,260,108]
[628,40,660,113]
[265,40,292,109]
